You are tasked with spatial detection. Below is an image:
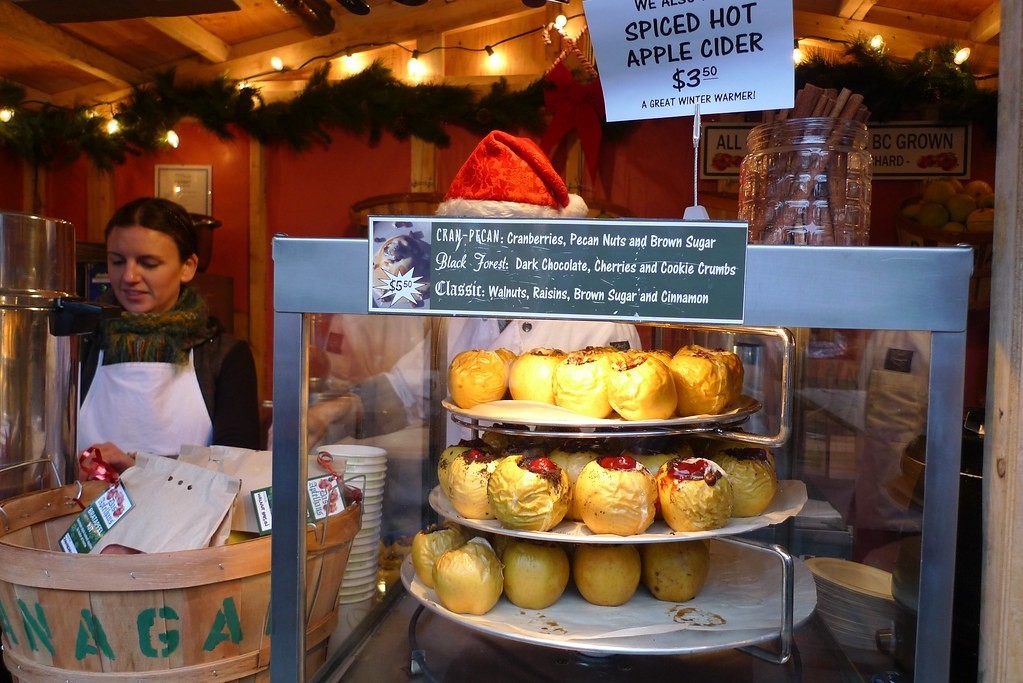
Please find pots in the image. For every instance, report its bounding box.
[191,212,223,272]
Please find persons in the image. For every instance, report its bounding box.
[34,198,262,491]
[267,133,644,546]
[843,327,931,565]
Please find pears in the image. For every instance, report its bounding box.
[902,177,998,234]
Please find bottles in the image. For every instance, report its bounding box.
[739,122,871,355]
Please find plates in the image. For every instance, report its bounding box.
[805,558,895,649]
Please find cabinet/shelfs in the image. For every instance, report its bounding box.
[400,326,811,683]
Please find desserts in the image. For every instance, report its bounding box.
[436,420,778,537]
[411,514,712,617]
[447,342,744,421]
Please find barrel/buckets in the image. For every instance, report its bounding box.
[893,198,994,317]
[0,479,362,683]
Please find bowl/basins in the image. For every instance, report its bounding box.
[317,446,387,664]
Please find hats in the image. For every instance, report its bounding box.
[437,130,588,220]
